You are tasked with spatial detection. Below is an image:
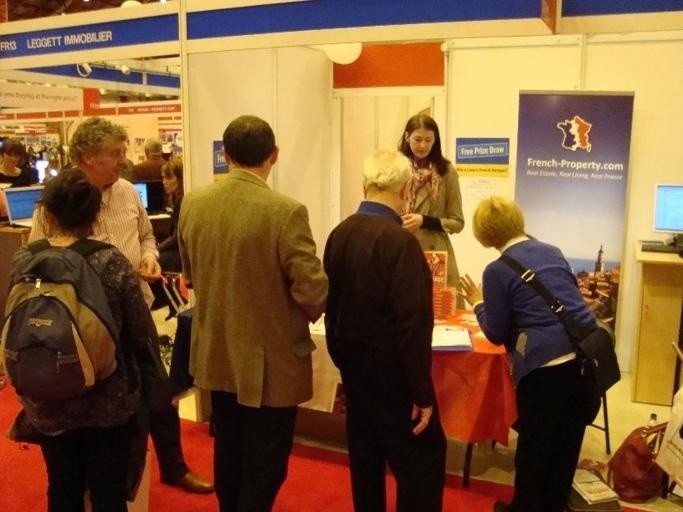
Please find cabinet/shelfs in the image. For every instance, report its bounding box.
[631,238,683,412]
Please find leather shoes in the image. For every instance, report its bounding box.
[160,471,214,492]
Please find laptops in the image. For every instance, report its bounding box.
[4,188,44,228]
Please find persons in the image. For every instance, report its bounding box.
[161,158,185,220]
[10,167,146,512]
[177,115,329,512]
[132,137,168,182]
[457,195,601,512]
[397,113,466,309]
[323,151,447,512]
[0,138,31,189]
[29,118,215,495]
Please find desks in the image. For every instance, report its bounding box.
[659,380,683,498]
[301,305,532,494]
[0,208,174,350]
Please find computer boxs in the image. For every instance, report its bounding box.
[144,181,164,211]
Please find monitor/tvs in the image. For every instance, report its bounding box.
[130,183,148,210]
[653,183,683,234]
[36,160,49,180]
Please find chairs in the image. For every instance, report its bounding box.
[580,316,622,458]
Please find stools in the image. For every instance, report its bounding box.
[160,273,190,317]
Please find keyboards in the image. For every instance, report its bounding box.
[641,244,682,253]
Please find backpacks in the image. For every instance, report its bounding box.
[0,239,122,401]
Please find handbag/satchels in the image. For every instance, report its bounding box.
[608,422,668,502]
[568,323,620,395]
